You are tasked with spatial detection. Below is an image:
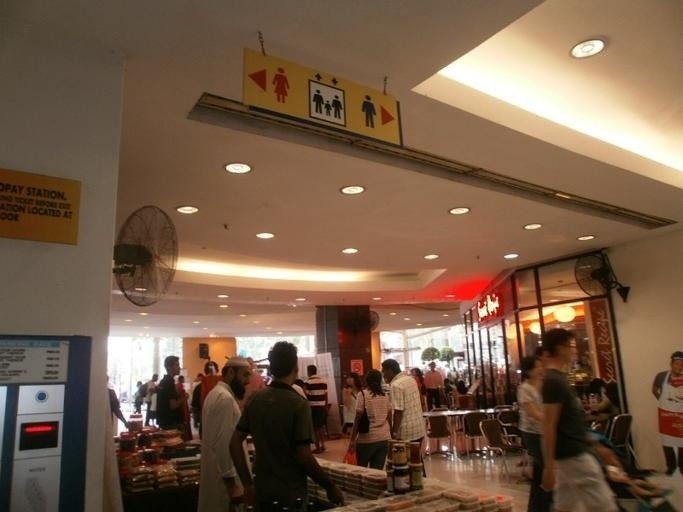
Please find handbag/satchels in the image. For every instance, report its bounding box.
[357,408,371,433]
[344,450,357,465]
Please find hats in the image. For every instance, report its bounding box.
[224,358,249,367]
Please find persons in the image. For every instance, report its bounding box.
[272,67,290,104]
[291,359,471,474]
[649,350,682,475]
[330,94,342,119]
[107,355,271,439]
[228,341,346,511]
[197,355,258,512]
[359,94,375,128]
[324,100,331,116]
[514,328,676,512]
[312,88,324,114]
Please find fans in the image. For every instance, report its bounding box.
[112,204,179,306]
[575,249,632,303]
[370,311,379,329]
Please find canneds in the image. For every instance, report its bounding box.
[386,439,423,495]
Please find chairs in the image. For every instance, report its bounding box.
[419,403,643,483]
